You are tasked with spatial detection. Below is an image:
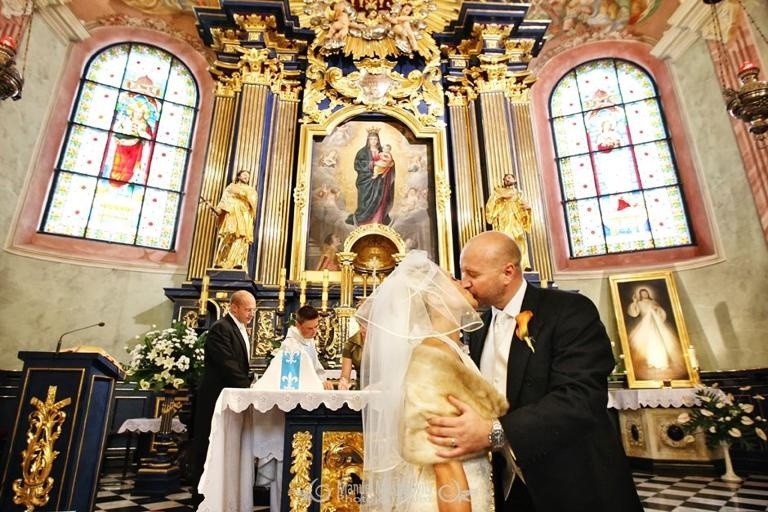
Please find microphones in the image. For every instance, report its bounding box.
[56,323,104,353]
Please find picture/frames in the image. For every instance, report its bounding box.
[289,107,454,284]
[608,269,701,389]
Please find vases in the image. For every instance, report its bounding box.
[148,385,177,468]
[718,440,742,482]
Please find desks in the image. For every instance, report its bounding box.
[196,387,366,512]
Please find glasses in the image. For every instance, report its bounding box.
[244,307,259,312]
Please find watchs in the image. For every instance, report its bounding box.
[489,420,506,452]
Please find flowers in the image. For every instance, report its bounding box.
[124,326,209,392]
[677,381,768,444]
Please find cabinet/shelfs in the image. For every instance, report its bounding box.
[0,350,129,512]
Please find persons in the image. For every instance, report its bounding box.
[345,128,395,226]
[485,173,532,272]
[180,290,256,512]
[425,230,644,511]
[628,289,686,378]
[324,2,362,42]
[338,300,376,389]
[361,251,509,512]
[372,144,393,178]
[281,306,334,390]
[211,171,258,269]
[382,3,420,54]
[362,8,381,26]
[318,234,343,271]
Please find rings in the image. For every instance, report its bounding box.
[451,438,456,447]
